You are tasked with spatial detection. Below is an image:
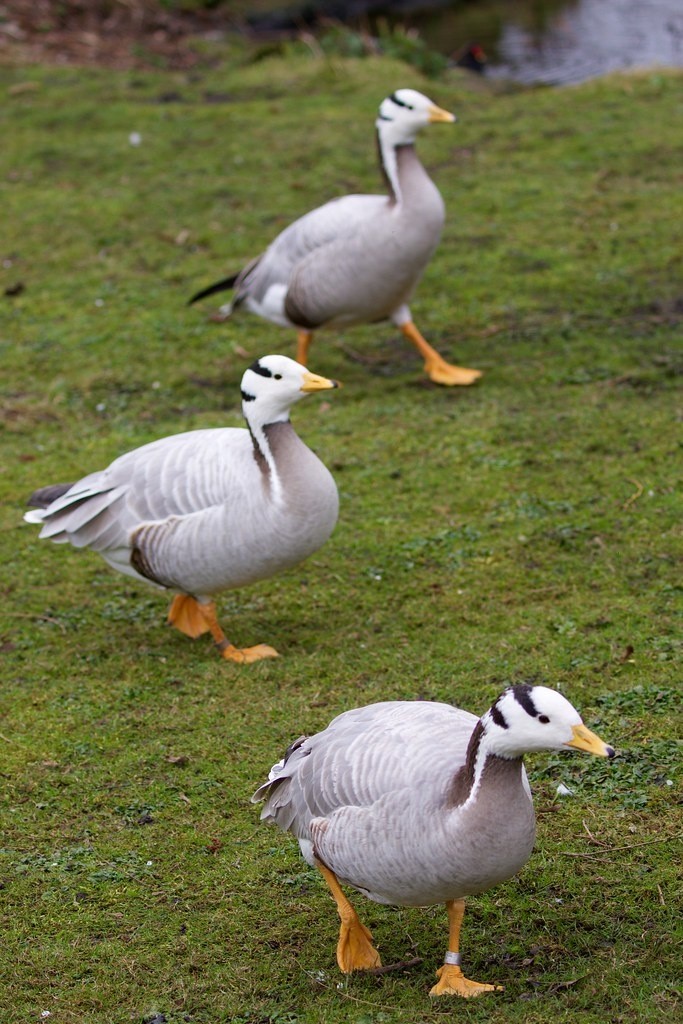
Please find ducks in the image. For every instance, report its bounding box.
[188,89,484,387]
[24,354,340,663]
[249,683,618,998]
